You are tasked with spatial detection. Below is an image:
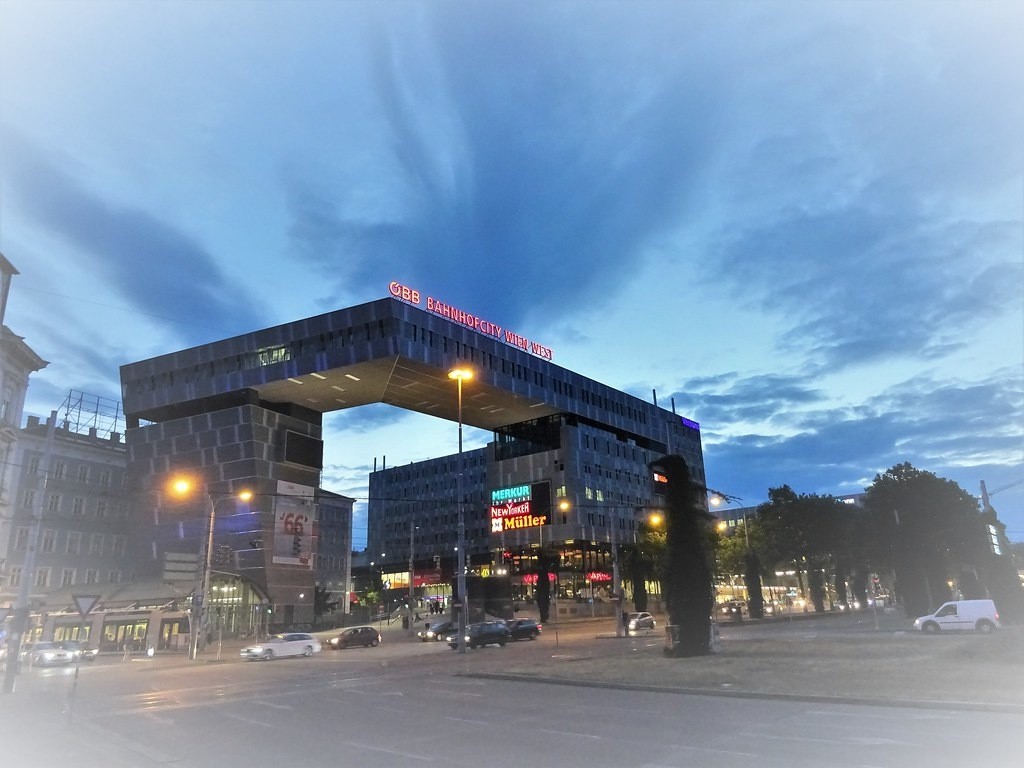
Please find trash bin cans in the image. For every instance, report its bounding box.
[425,623,430,630]
[709,623,721,653]
[664,625,680,651]
[402,617,408,629]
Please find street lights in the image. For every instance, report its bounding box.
[539,501,569,547]
[166,476,256,651]
[448,367,474,653]
[712,496,749,550]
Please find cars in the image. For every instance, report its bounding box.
[326,626,382,650]
[718,591,890,621]
[20,639,99,666]
[239,633,323,661]
[418,621,459,641]
[628,612,655,631]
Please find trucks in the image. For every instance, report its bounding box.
[913,599,1001,633]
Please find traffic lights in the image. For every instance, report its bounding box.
[653,466,671,496]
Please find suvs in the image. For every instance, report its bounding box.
[504,618,543,641]
[445,620,508,650]
